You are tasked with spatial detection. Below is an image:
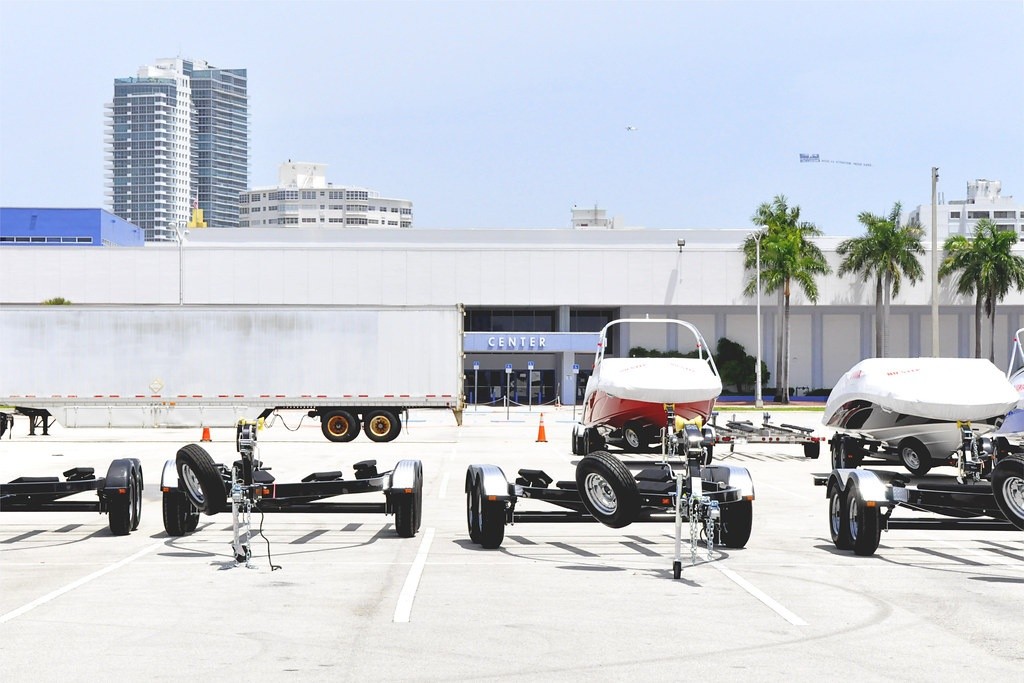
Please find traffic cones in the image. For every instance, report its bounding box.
[535,412,548,442]
[198,426,213,442]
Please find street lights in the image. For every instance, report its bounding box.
[167,222,191,304]
[931,166,943,359]
[746,223,771,408]
